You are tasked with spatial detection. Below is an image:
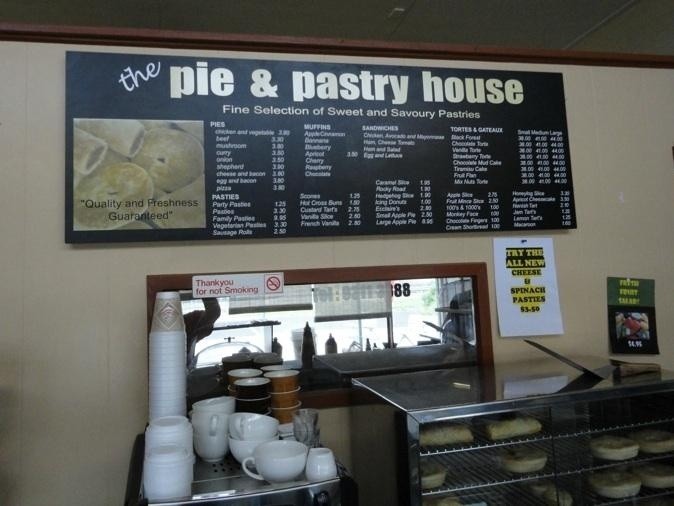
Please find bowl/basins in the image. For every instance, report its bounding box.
[141,445,192,500]
[143,417,194,445]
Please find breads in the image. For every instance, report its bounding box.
[526,478,555,494]
[420,461,448,490]
[485,417,542,442]
[629,429,674,453]
[590,470,642,498]
[633,464,674,488]
[499,446,548,473]
[545,488,575,506]
[419,421,474,446]
[590,435,640,460]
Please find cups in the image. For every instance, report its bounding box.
[223,355,250,368]
[147,292,187,422]
[269,391,299,409]
[264,371,299,394]
[243,440,305,483]
[230,413,277,440]
[227,438,274,468]
[259,364,287,373]
[253,352,282,365]
[228,368,262,384]
[195,397,235,415]
[237,397,269,413]
[228,380,272,399]
[191,413,229,434]
[291,409,318,445]
[194,436,229,462]
[274,407,299,424]
[305,448,337,483]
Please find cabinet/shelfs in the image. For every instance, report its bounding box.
[349,359,674,502]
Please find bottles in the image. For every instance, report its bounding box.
[325,333,337,354]
[302,321,315,367]
[272,337,282,356]
